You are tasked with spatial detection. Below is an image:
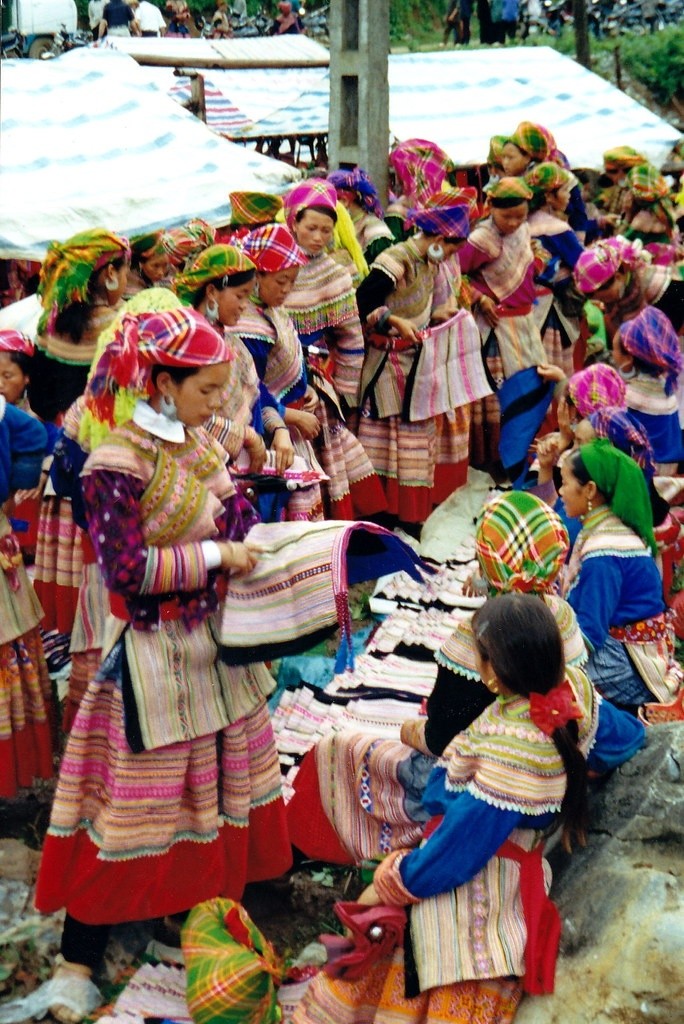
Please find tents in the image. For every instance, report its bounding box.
[1,31,684,264]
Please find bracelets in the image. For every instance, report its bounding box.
[226,539,236,570]
[247,434,264,452]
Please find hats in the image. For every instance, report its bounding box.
[228,191,283,231]
[602,145,646,171]
[487,136,514,168]
[485,177,534,200]
[525,162,578,196]
[504,121,556,162]
[474,490,570,597]
[619,303,683,398]
[282,178,338,232]
[624,161,672,203]
[407,206,470,239]
[563,363,628,421]
[422,186,479,220]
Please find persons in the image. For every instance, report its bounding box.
[269,1,301,36]
[0,128,683,1024]
[442,0,520,46]
[85,0,234,48]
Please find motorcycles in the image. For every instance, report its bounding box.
[538,0,683,39]
[196,0,330,40]
[0,0,95,61]
